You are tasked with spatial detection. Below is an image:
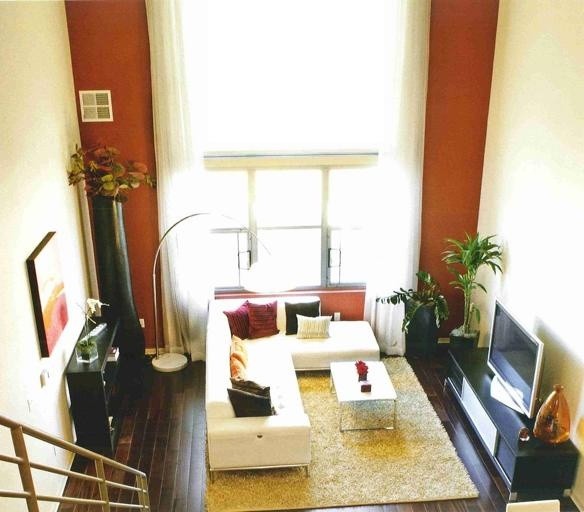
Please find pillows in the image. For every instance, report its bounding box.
[222,301,333,416]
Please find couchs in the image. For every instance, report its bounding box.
[205,295,380,485]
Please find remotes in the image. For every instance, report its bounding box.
[79,322,107,342]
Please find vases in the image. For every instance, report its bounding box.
[90,195,129,204]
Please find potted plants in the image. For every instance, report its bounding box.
[70,295,110,363]
[440,230,512,351]
[373,271,450,371]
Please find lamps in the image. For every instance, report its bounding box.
[149,210,290,374]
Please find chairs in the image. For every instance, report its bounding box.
[504,499,562,512]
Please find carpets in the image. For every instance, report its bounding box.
[203,354,481,511]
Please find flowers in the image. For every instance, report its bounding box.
[66,138,155,194]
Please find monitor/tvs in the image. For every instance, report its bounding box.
[486,298,544,419]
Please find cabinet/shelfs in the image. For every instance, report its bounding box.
[62,316,131,464]
